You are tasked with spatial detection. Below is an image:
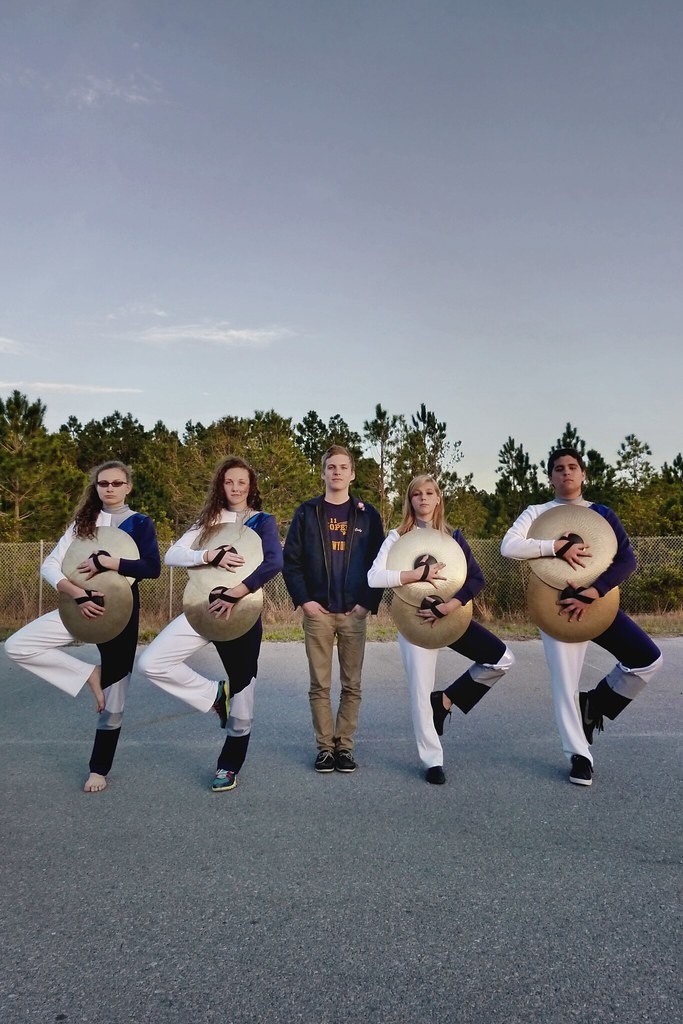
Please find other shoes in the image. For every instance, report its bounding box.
[314,750,335,772]
[430,691,452,736]
[334,749,359,772]
[425,766,446,785]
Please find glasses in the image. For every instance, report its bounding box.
[96,480,128,488]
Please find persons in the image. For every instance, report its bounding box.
[138,452,285,791]
[499,447,663,786]
[281,443,384,776]
[366,473,515,786]
[5,460,162,794]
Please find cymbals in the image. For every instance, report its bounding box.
[59,527,141,588]
[181,568,265,643]
[525,570,621,644]
[389,593,474,650]
[57,567,134,645]
[187,522,266,578]
[524,505,618,588]
[386,528,469,609]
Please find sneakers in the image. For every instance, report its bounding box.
[574,691,605,746]
[212,769,237,791]
[569,753,595,787]
[212,680,232,729]
